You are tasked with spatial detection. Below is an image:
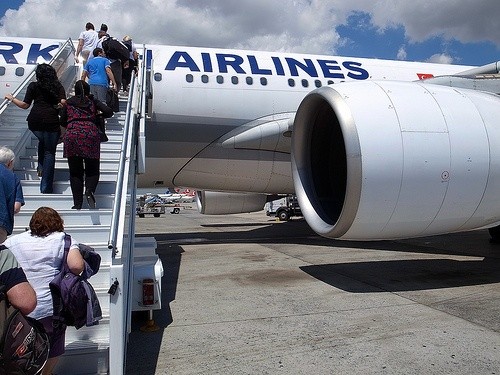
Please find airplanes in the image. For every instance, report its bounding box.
[0,35,500,243]
[134,193,196,204]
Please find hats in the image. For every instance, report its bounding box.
[123,36,132,41]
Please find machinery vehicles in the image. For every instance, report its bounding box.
[264,194,303,221]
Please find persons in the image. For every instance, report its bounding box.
[3,207,84,375]
[3,63,67,194]
[75,22,138,104]
[0,146,25,235]
[59,80,114,209]
[0,228,37,375]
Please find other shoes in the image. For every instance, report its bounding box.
[85,191,97,209]
[72,204,82,209]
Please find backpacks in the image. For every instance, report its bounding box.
[51,233,88,330]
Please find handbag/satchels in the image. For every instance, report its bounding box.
[106,89,120,113]
[0,294,49,375]
[102,36,130,61]
[97,116,109,141]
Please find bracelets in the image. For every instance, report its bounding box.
[12,97,15,102]
